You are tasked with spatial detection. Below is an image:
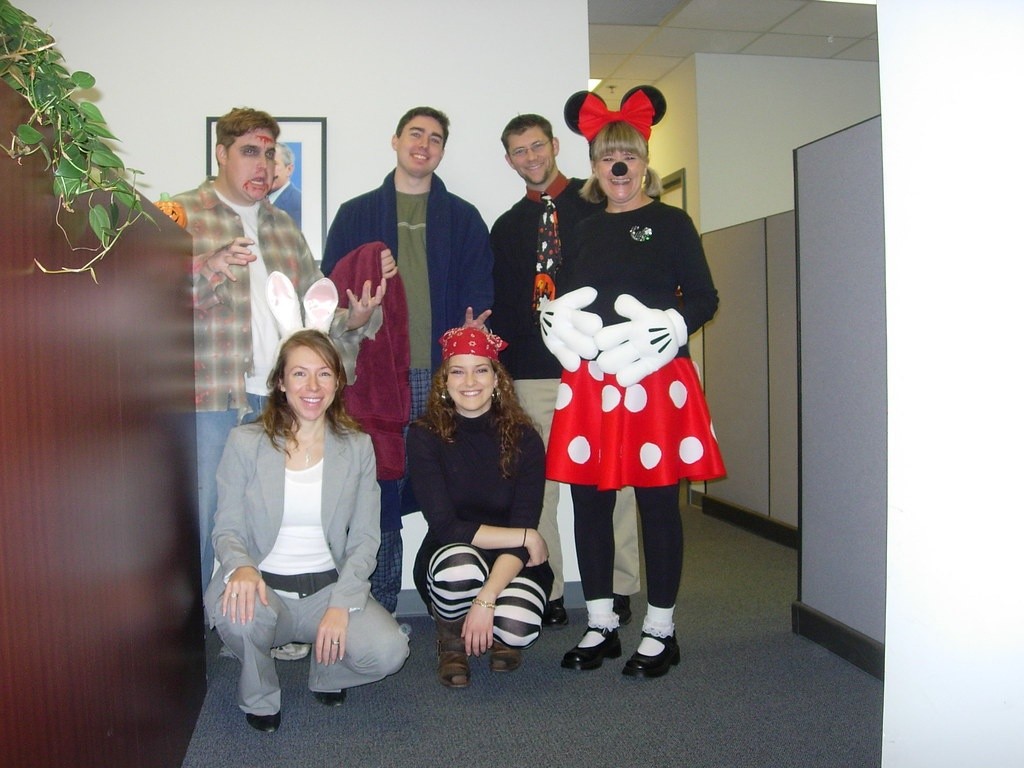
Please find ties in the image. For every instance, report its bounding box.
[532,191,563,329]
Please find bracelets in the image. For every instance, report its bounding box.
[472,599,495,610]
[522,528,526,547]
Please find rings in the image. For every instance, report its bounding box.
[231,593,237,597]
[332,641,339,645]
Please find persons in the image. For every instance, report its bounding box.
[490,114,641,628]
[201,329,410,733]
[405,328,555,688]
[539,84,727,679]
[320,107,494,618]
[172,107,387,661]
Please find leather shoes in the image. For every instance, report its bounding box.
[613,592,631,626]
[622,631,680,678]
[542,598,568,628]
[315,689,344,707]
[246,710,281,732]
[561,626,623,671]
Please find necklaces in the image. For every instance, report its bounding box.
[305,452,312,464]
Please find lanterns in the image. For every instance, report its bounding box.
[153,200,188,229]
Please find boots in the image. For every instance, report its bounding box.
[489,638,521,673]
[430,601,469,687]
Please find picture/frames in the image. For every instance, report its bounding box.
[206,117,327,265]
